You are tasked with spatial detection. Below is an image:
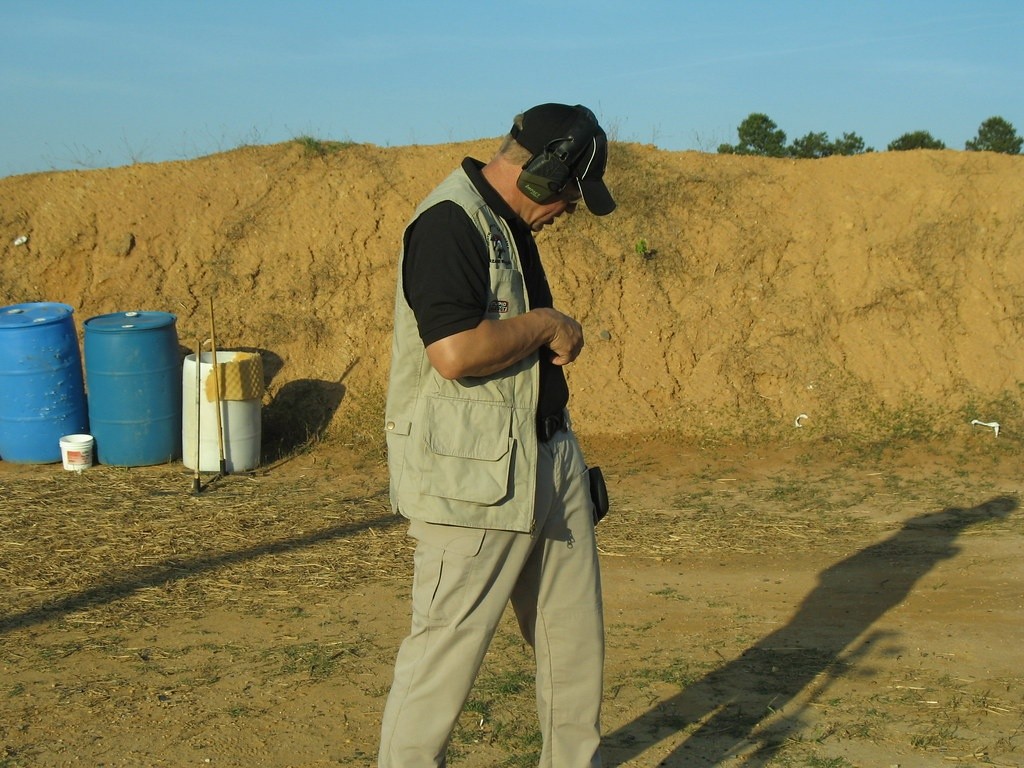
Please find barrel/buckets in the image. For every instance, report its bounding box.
[0,302,86,466]
[81,311,185,467]
[59,434,94,471]
[183,352,264,471]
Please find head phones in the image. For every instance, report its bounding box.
[517,105,598,204]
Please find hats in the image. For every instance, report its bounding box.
[511,103,616,217]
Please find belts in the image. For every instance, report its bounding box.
[536,412,565,444]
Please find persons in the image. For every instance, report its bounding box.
[374,103,616,768]
